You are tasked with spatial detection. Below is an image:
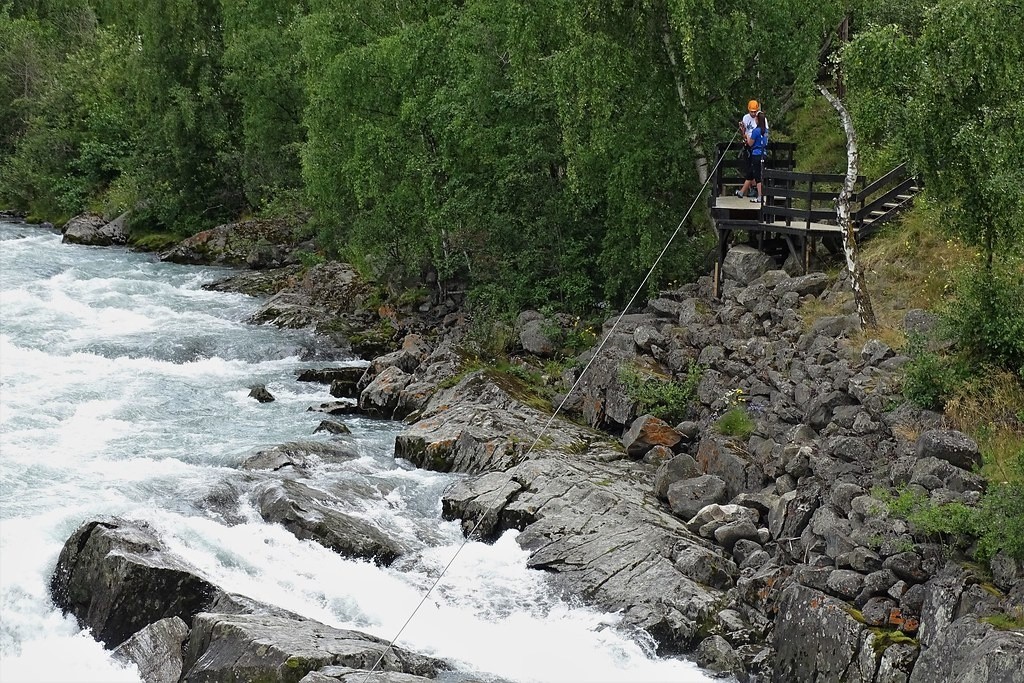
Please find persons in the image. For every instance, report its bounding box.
[733,111,768,203]
[738,100,769,197]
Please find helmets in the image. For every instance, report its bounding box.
[747,100,758,111]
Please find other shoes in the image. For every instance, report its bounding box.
[734,190,743,198]
[750,198,761,203]
